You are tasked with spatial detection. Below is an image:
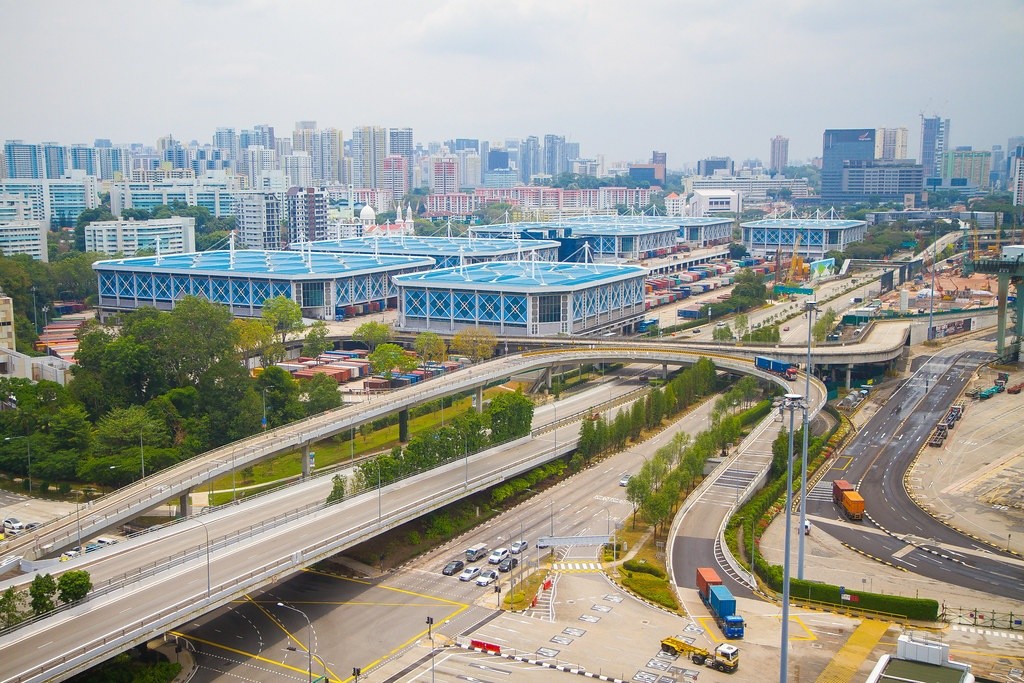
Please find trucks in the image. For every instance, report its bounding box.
[465,543,488,562]
[639,320,653,333]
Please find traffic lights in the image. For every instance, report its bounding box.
[352,667,356,676]
[426,617,429,624]
[430,618,433,624]
[356,668,361,676]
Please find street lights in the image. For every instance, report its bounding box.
[797,301,822,579]
[771,393,810,683]
[192,518,210,597]
[287,647,328,683]
[526,489,554,556]
[491,509,522,587]
[277,603,311,683]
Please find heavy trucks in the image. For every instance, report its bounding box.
[696,567,747,639]
[979,372,1010,399]
[660,635,739,674]
[755,356,797,381]
[942,406,962,428]
[831,480,864,521]
[927,423,948,447]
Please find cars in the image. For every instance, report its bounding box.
[509,541,528,554]
[488,548,509,564]
[459,567,481,582]
[536,535,553,549]
[498,557,518,572]
[0,518,117,558]
[476,569,499,586]
[442,561,464,576]
